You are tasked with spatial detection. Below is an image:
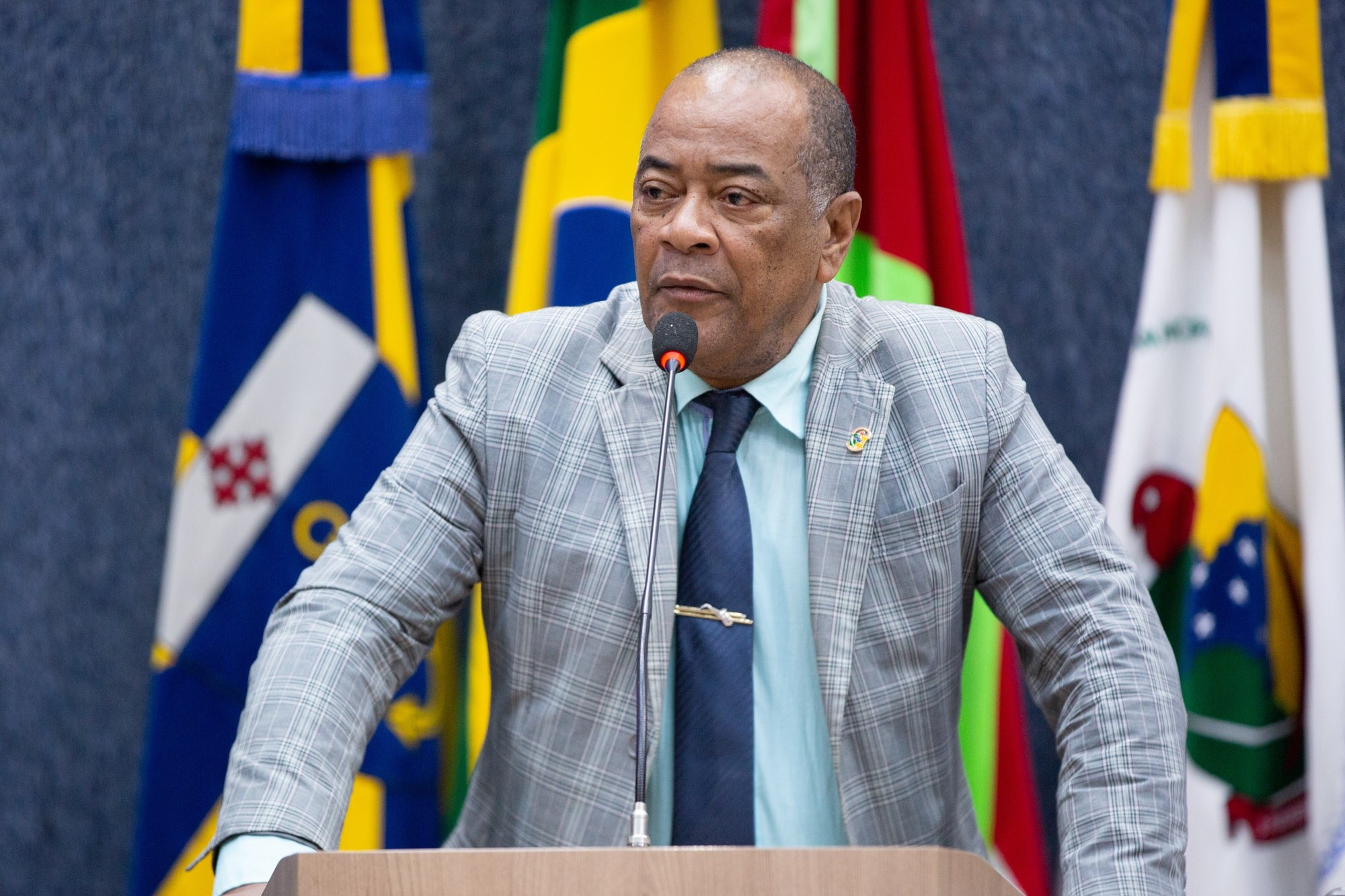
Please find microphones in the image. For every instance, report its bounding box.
[628,312,697,845]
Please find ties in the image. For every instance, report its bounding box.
[672,389,765,845]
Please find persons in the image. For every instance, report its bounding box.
[182,47,1190,895]
